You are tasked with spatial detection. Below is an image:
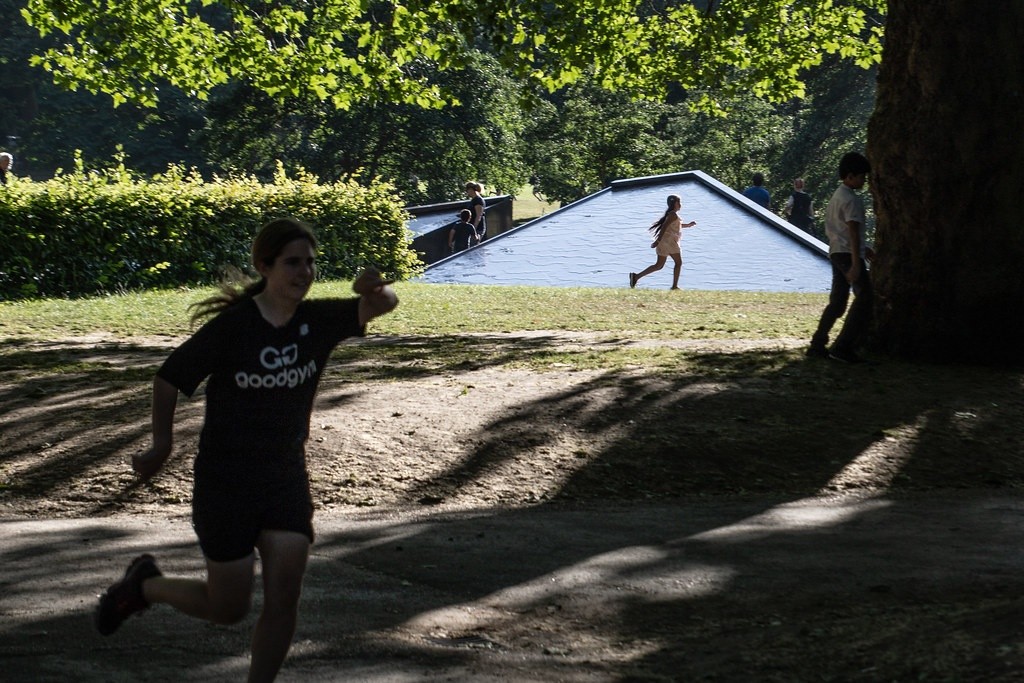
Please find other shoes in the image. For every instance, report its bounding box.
[95,553,163,635]
[806,339,829,354]
[630,272,637,289]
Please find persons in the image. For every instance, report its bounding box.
[785,178,814,233]
[448,183,487,253]
[805,152,875,365]
[94,218,400,683]
[630,195,696,290]
[743,173,771,211]
[0,152,13,187]
